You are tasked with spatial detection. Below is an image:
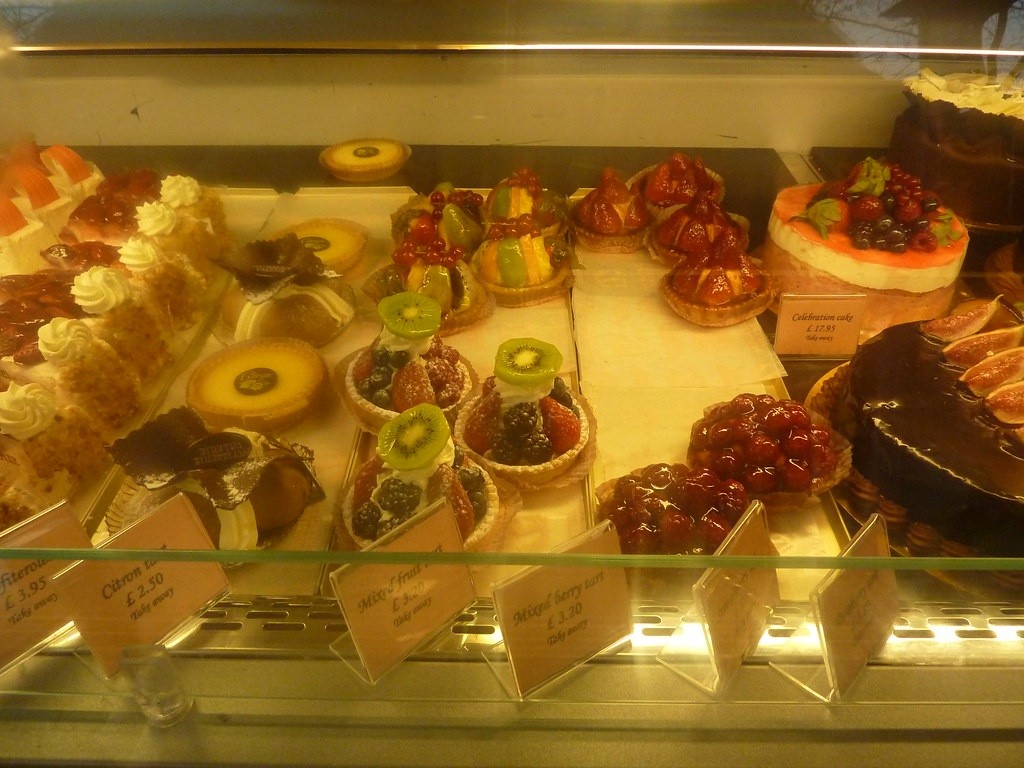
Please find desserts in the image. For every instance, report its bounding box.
[0,139,857,570]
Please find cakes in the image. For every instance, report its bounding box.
[813,291,1024,594]
[889,68,1024,229]
[756,155,970,344]
